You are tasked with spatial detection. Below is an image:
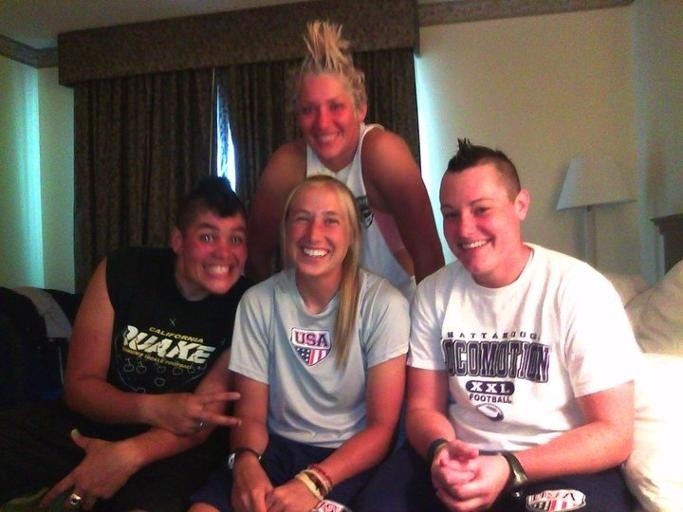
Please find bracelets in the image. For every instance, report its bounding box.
[307,460,333,492]
[293,470,330,502]
[426,436,448,464]
[225,445,262,471]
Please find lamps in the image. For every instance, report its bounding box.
[555,155,637,269]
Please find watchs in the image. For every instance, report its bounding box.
[499,451,530,508]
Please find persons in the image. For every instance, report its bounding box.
[239,13,446,302]
[185,170,426,512]
[36,171,263,512]
[401,134,642,512]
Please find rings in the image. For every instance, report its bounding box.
[67,493,83,508]
[198,420,209,433]
